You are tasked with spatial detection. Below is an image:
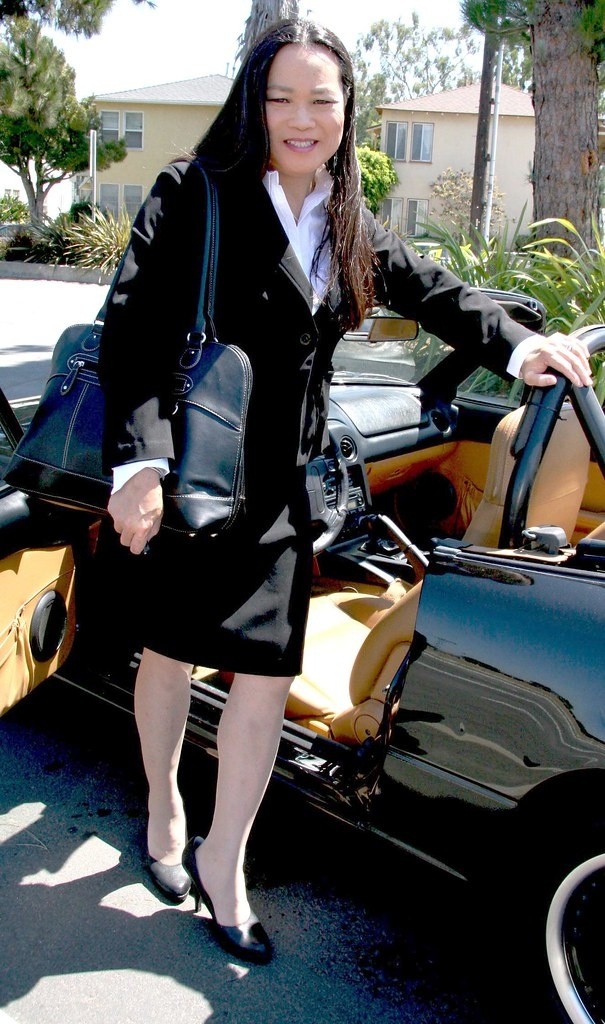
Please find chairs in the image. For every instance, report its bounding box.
[217,403,590,749]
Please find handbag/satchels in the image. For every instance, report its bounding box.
[2,157,252,538]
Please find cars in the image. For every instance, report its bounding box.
[0,223,65,261]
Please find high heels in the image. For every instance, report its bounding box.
[182,835,272,966]
[145,854,191,902]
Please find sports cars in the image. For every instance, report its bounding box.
[0,285,605,1024]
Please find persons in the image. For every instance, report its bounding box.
[99,18,595,968]
